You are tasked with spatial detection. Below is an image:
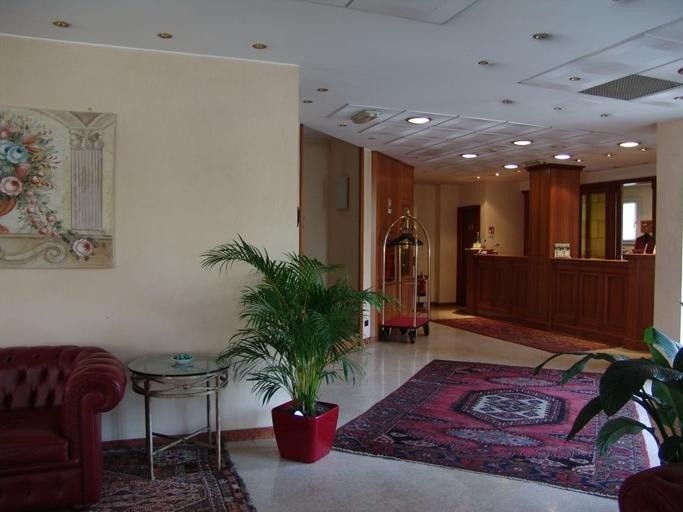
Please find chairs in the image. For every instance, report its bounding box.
[0,344,126,511]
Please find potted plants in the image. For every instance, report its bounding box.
[195,232,401,464]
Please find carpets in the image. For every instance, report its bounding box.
[36,441,256,512]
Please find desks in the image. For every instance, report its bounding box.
[128,351,232,481]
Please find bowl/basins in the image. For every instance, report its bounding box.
[171,353,192,363]
